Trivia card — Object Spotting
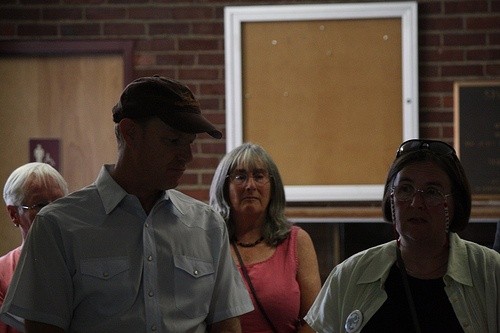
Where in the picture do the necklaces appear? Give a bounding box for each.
[233,232,266,248]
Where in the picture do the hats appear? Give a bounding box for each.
[112,74,223,139]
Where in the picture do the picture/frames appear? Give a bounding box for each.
[224,1,418,203]
[454,81,499,192]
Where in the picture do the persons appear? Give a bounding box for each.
[1,77,253,332]
[304,140,499,333]
[209,144,321,332]
[0,162,68,333]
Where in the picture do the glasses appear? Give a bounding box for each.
[394,139,464,184]
[226,172,272,185]
[16,202,49,213]
[391,185,456,207]
